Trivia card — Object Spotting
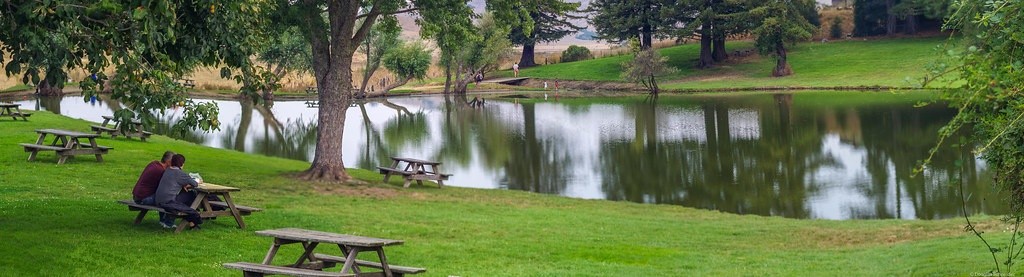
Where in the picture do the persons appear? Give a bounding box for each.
[545,81,547,89]
[133,151,202,229]
[555,81,558,90]
[513,62,519,78]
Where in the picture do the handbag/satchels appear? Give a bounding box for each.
[189,173,203,184]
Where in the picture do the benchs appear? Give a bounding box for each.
[89,125,118,131]
[20,143,71,151]
[313,253,426,273]
[174,82,196,86]
[118,201,189,215]
[14,112,31,115]
[306,89,318,92]
[419,171,454,177]
[223,262,358,277]
[208,201,263,212]
[74,143,114,150]
[129,128,154,134]
[377,167,411,175]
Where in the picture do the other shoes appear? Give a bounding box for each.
[159,222,177,229]
[190,224,201,230]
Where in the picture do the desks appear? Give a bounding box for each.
[244,228,406,277]
[172,183,246,234]
[0,103,27,121]
[96,116,146,142]
[172,76,194,90]
[28,129,103,164]
[383,157,443,188]
[307,87,318,93]
